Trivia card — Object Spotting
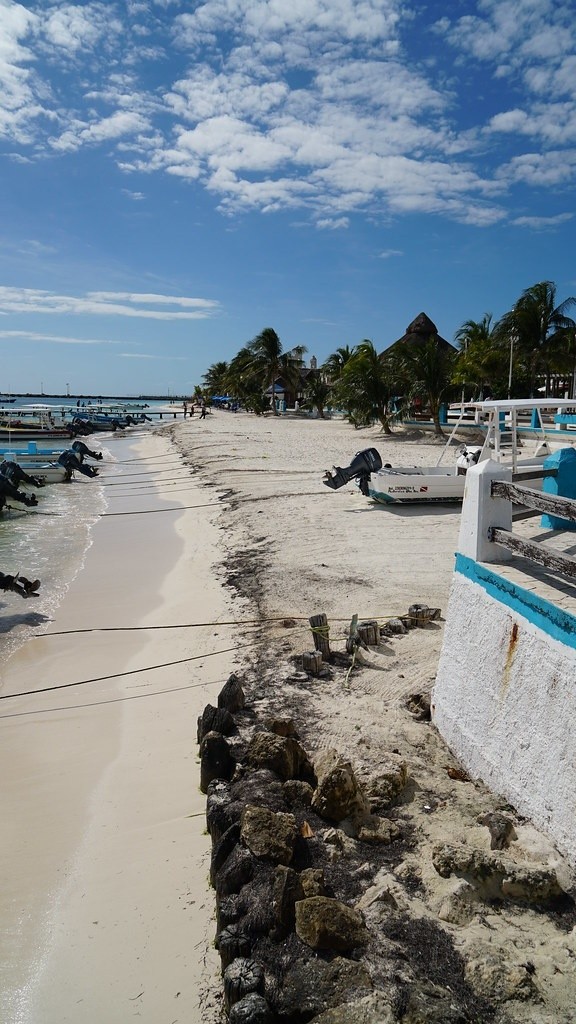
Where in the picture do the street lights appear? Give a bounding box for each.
[508,326,519,400]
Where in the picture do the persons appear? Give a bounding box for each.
[76,400,237,419]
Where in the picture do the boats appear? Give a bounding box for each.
[0,461,45,511]
[321,398,576,505]
[0,384,103,482]
[0,400,152,441]
[0,398,17,403]
